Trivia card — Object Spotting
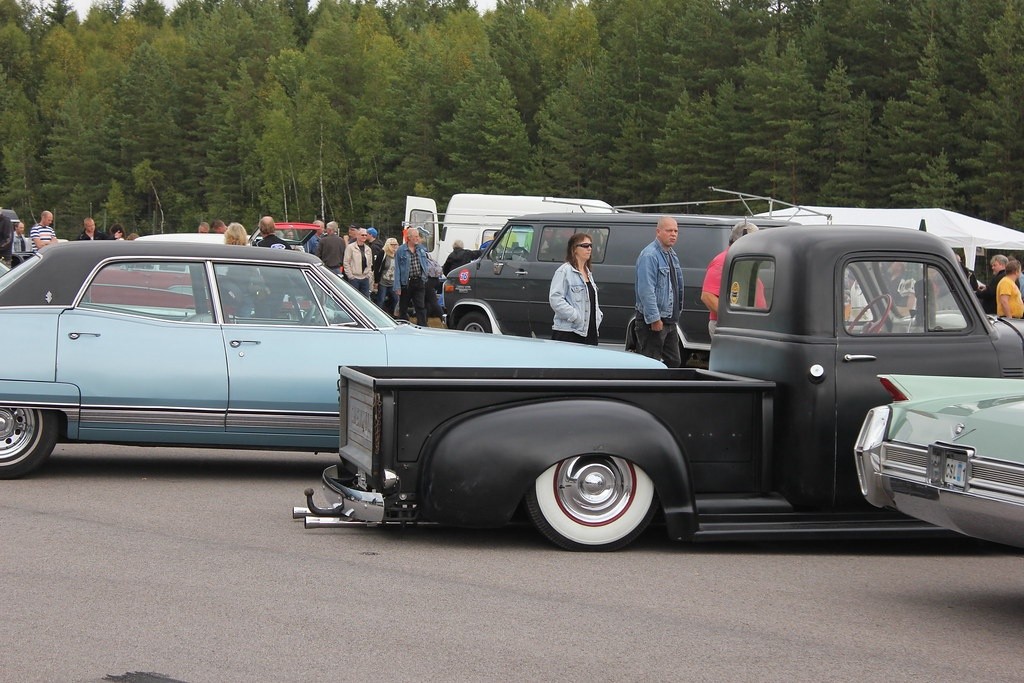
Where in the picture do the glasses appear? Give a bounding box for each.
[361,234,367,237]
[575,243,593,248]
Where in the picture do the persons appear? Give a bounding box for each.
[78,218,139,241]
[443,239,482,280]
[199,222,210,233]
[373,237,399,316]
[224,223,248,245]
[0,209,14,264]
[12,221,30,268]
[213,220,228,234]
[996,261,1024,319]
[254,216,292,250]
[303,220,384,300]
[635,217,684,368]
[30,210,58,255]
[394,228,428,327]
[1007,255,1024,300]
[701,222,767,338]
[845,255,978,321]
[549,233,603,346]
[978,254,1020,315]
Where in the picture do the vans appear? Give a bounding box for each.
[440,210,804,369]
[401,192,617,292]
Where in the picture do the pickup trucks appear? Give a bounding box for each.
[291,221,1024,552]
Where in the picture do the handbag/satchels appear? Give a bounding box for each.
[369,271,378,293]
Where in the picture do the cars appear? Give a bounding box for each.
[83,231,250,311]
[853,373,1024,555]
[248,221,345,313]
[0,239,672,481]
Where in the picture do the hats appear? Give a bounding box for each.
[366,228,378,238]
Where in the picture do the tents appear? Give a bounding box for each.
[755,206,1024,272]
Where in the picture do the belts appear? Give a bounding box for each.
[410,277,421,281]
